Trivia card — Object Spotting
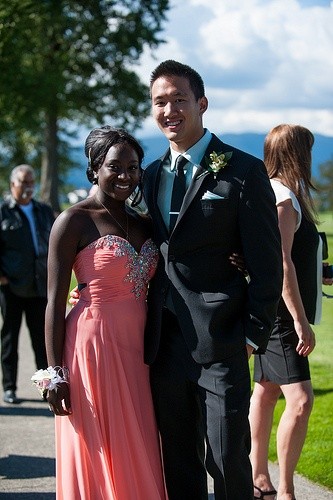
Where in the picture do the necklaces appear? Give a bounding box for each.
[94,192,131,245]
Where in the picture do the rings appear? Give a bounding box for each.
[308,344,312,347]
[299,340,303,344]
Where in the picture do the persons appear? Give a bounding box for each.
[246,124,333,500]
[69,59,283,500]
[0,161,62,405]
[32,125,250,500]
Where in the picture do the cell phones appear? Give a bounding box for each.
[323,265,333,278]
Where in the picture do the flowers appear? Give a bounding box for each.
[206,151,232,180]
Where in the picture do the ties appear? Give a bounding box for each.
[168,156,190,236]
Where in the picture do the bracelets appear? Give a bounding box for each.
[29,365,68,400]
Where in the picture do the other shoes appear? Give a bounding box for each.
[4,389,17,404]
[254,482,277,500]
